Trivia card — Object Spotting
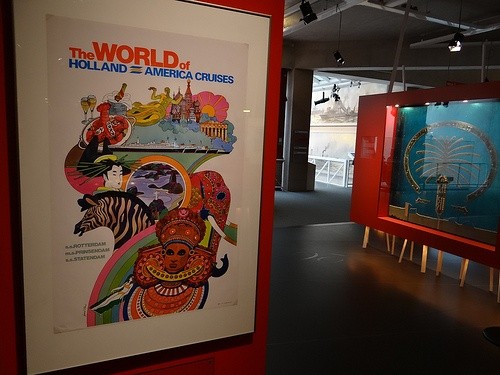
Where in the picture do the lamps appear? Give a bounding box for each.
[333,4,345,65]
[447,0,465,53]
[299,0,317,27]
